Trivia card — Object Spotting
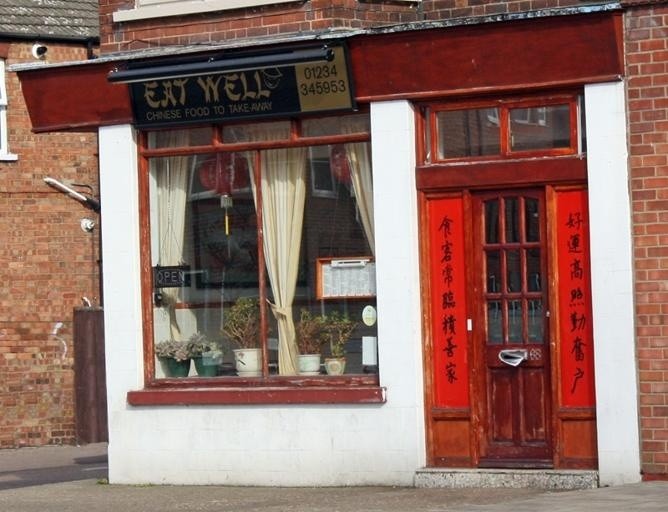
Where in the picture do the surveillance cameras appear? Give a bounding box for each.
[32,43,48,58]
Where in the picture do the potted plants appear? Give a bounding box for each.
[156,294,363,376]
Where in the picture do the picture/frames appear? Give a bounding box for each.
[314,255,376,303]
